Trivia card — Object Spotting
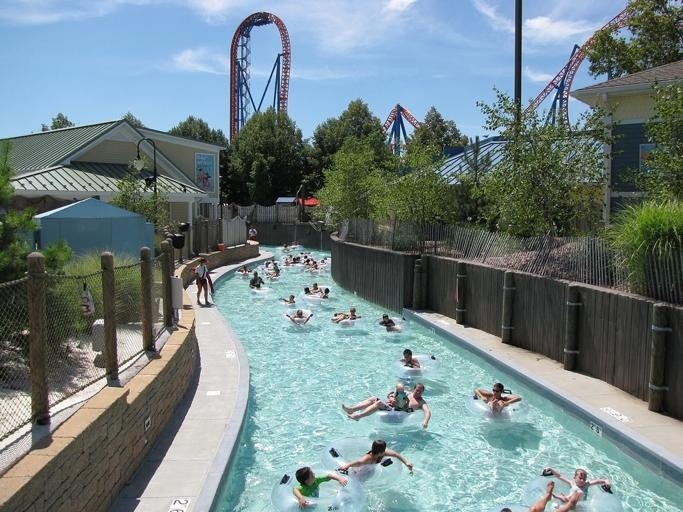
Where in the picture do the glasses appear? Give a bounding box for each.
[493,389,499,392]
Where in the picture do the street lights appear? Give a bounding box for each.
[133,136,158,235]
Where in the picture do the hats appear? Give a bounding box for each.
[199,259,207,263]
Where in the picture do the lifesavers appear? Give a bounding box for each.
[335,311,365,328]
[278,297,305,308]
[374,316,408,336]
[320,436,403,490]
[393,353,440,380]
[490,503,533,512]
[519,470,623,511]
[270,466,369,512]
[360,389,428,437]
[301,285,335,306]
[236,245,329,295]
[464,388,521,420]
[283,307,316,330]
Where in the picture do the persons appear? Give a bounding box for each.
[284,310,315,324]
[191,258,214,305]
[379,313,400,333]
[332,307,362,325]
[237,241,330,305]
[248,225,258,241]
